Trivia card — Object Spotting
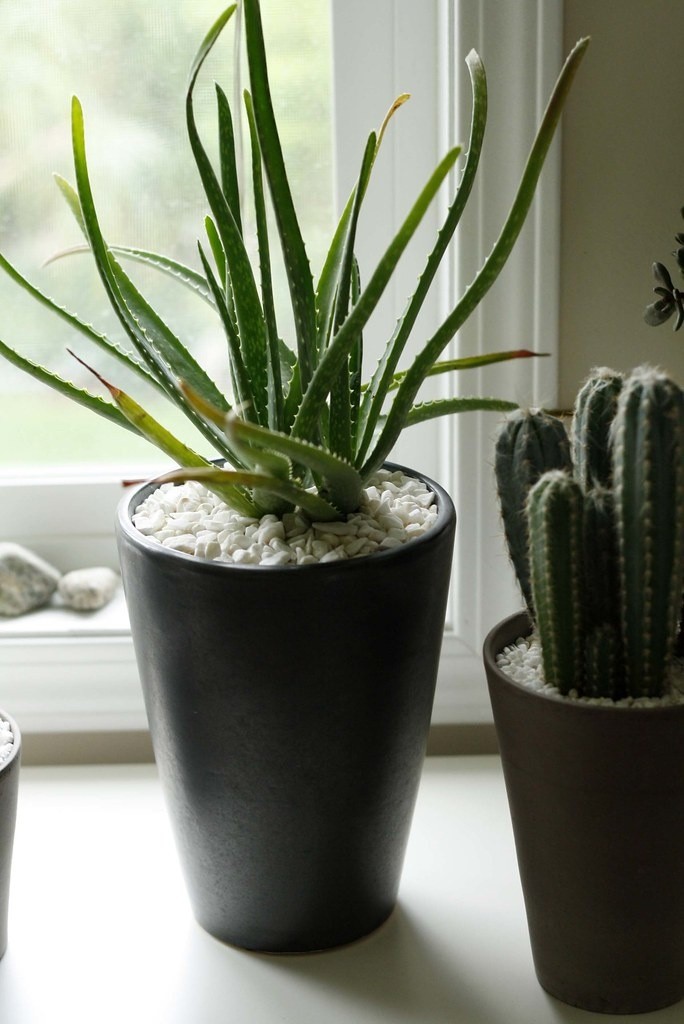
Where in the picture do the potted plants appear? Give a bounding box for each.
[476,351,684,1009]
[1,0,595,955]
